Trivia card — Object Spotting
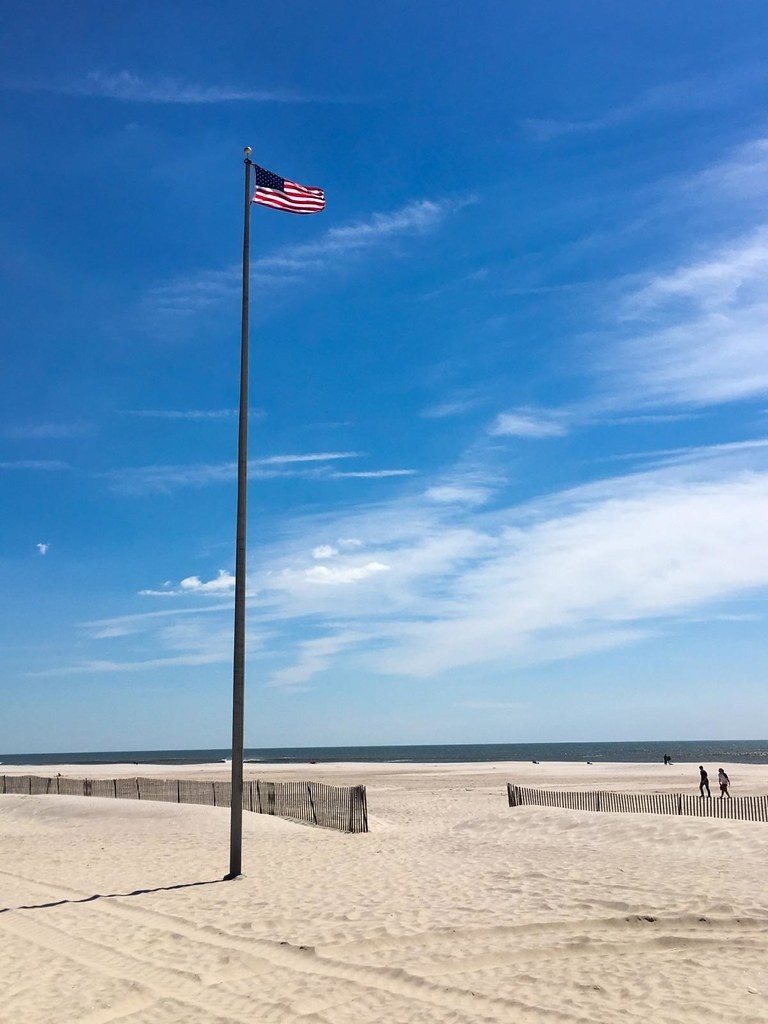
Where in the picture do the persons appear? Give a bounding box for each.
[699,765,711,798]
[663,753,672,765]
[718,768,732,800]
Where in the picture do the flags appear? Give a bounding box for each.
[250,162,326,215]
